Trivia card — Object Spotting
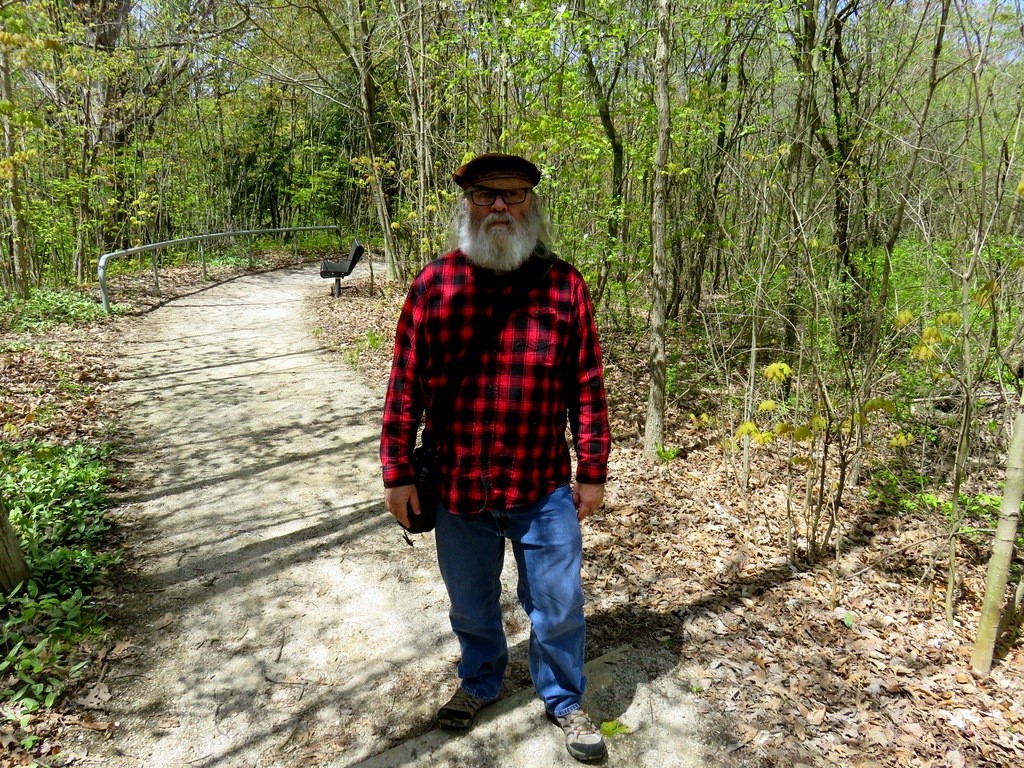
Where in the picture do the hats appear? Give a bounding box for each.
[452,154,541,195]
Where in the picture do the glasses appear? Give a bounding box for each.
[466,188,534,206]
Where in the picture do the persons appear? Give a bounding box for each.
[380,151,612,763]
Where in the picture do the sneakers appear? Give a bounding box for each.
[437,686,504,728]
[546,708,604,760]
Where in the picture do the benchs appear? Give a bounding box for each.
[319,238,365,298]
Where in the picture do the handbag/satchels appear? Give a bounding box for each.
[396,447,437,534]
